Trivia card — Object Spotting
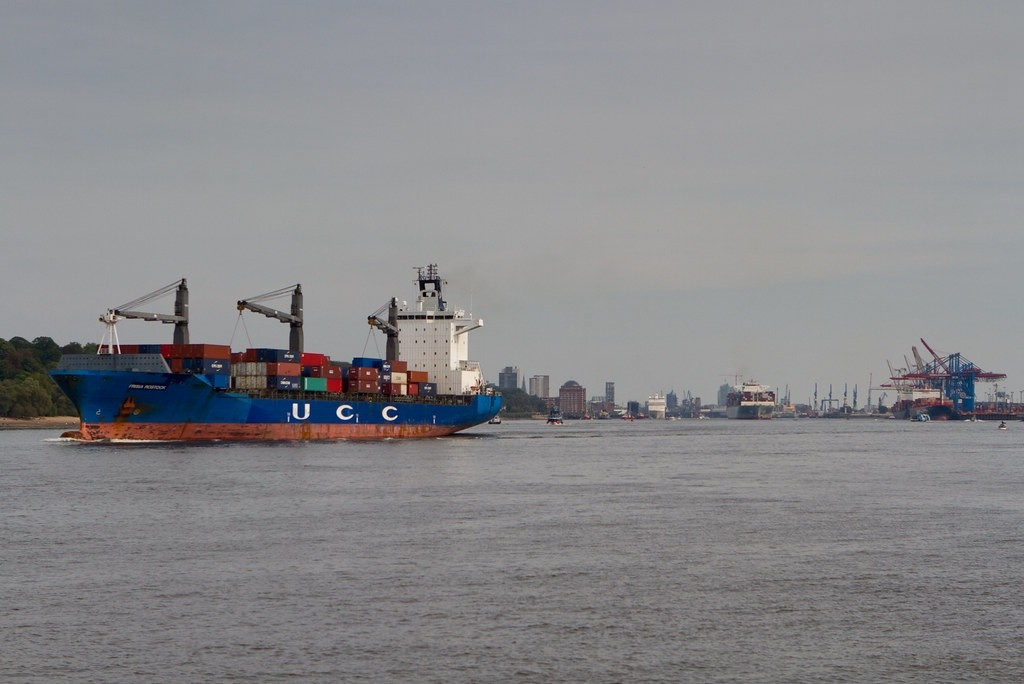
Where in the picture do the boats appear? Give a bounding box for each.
[726,378,775,420]
[891,399,916,420]
[597,410,610,419]
[912,411,930,422]
[488,416,502,424]
[799,414,808,417]
[909,397,954,420]
[696,413,710,420]
[964,414,984,423]
[547,404,564,424]
[772,403,796,418]
[583,414,590,419]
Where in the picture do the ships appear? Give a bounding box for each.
[49,263,506,439]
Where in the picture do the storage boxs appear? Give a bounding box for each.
[97,343,438,397]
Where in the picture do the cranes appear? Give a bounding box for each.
[722,373,744,386]
